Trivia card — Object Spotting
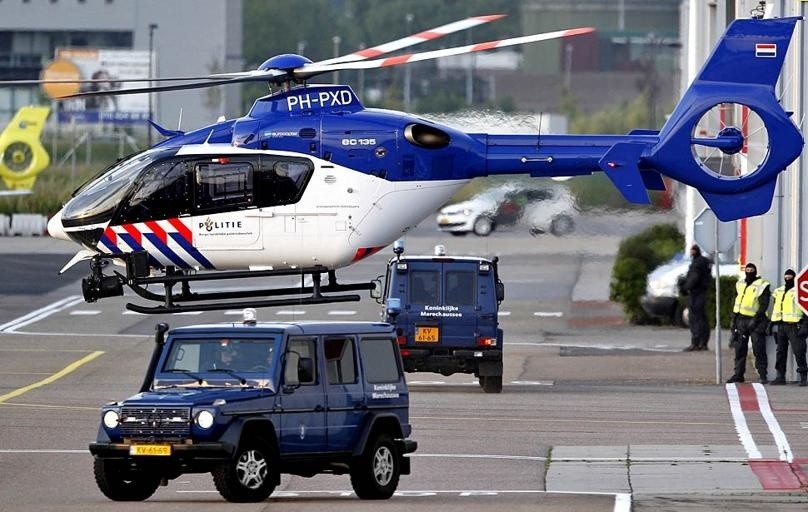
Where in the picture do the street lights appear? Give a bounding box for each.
[148,22,159,147]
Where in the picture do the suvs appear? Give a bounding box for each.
[370,240,504,393]
[84,320,417,503]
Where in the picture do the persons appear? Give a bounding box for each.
[726,263,772,384]
[676,244,717,352]
[83,69,119,111]
[197,340,242,373]
[768,267,808,387]
[261,345,312,385]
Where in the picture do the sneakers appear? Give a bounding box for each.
[797,379,807,387]
[755,375,768,384]
[725,373,744,383]
[769,376,787,387]
[682,344,709,352]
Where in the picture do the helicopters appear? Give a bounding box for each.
[1,15,803,315]
[0,105,51,191]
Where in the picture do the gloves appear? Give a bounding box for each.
[765,321,773,336]
[729,316,737,331]
[796,319,808,339]
[746,319,757,331]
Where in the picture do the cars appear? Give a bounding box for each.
[638,232,741,329]
[436,183,578,236]
